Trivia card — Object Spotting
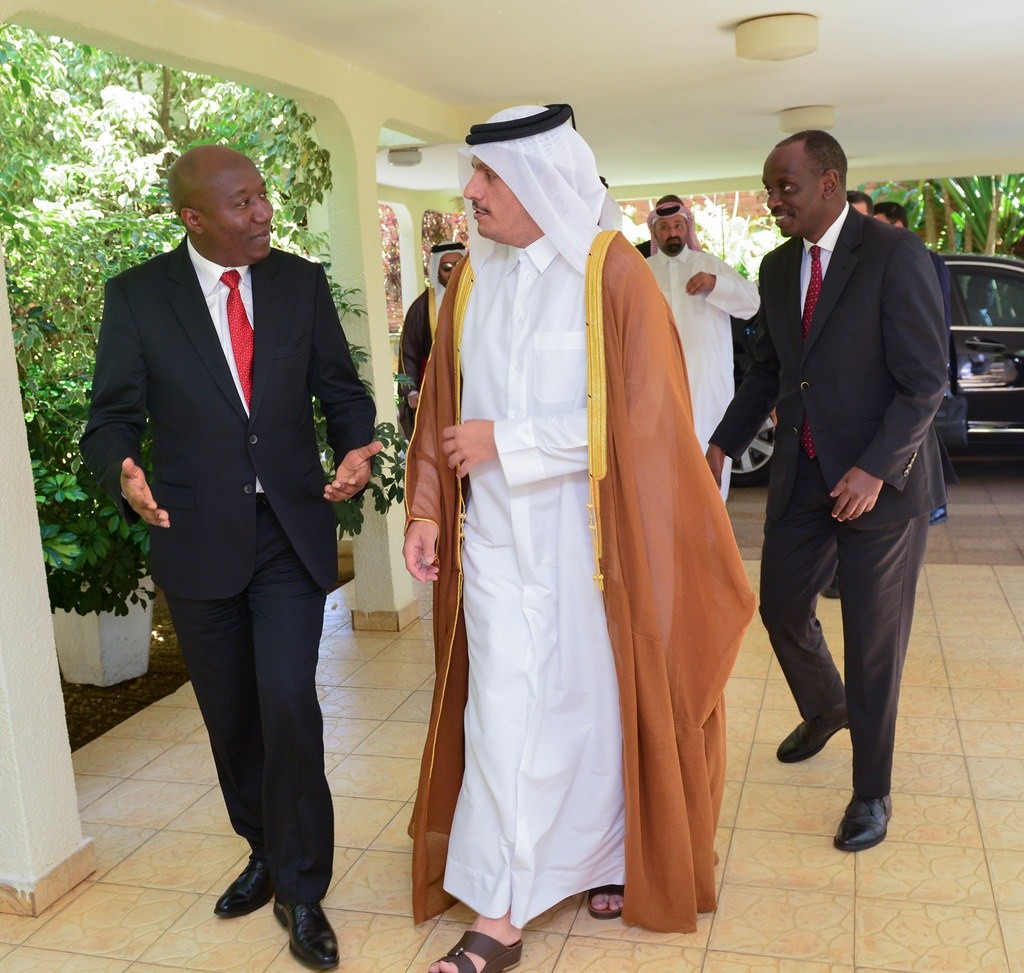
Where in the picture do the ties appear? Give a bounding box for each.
[220,270,255,410]
[801,243,824,462]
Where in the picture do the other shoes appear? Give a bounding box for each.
[928,503,947,525]
[820,570,840,598]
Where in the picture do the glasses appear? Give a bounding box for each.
[439,260,457,272]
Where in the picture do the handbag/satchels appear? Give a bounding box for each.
[932,387,971,447]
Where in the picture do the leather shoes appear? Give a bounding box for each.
[214,858,274,919]
[776,715,850,763]
[272,900,339,969]
[833,793,893,851]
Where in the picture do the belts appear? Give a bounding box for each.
[257,494,268,505]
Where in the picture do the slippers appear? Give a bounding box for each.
[425,931,523,973]
[588,883,626,920]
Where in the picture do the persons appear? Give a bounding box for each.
[400,106,754,973]
[819,191,953,599]
[641,193,762,508]
[967,275,998,326]
[78,145,384,971]
[707,128,950,853]
[398,241,468,440]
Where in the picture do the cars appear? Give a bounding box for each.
[728,253,1024,485]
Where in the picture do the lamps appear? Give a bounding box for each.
[779,104,839,134]
[736,14,818,60]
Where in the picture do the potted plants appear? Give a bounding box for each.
[9,306,154,687]
